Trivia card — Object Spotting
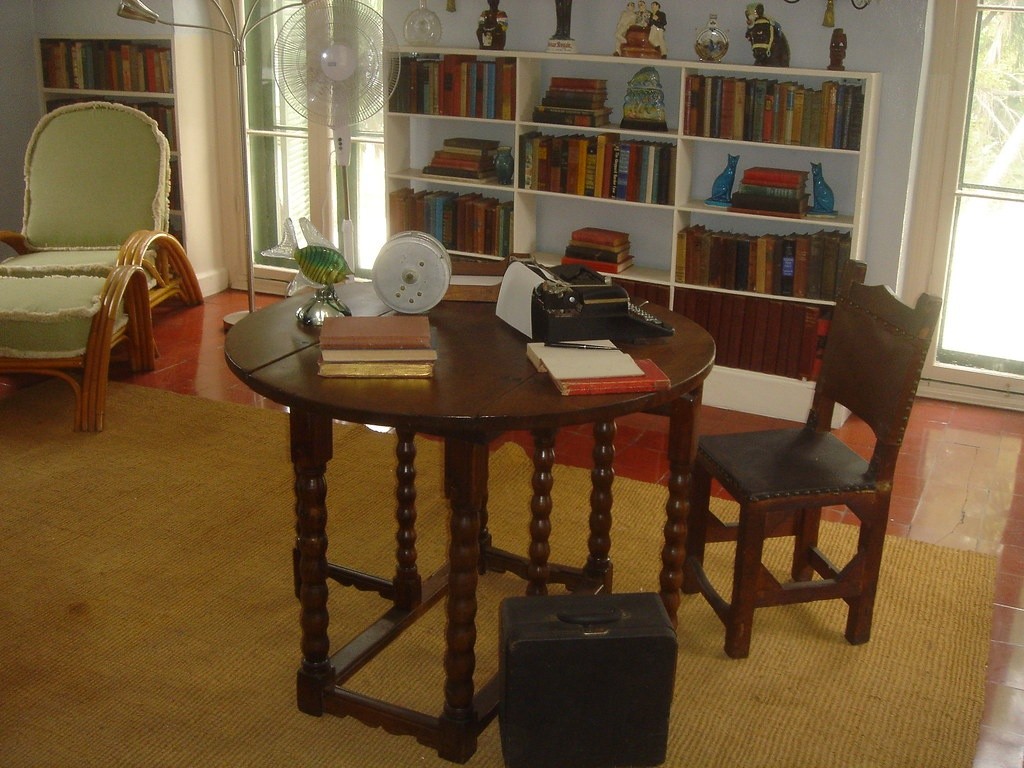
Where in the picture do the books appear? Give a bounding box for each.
[674,289,834,382]
[422,138,500,184]
[526,339,671,396]
[318,317,436,377]
[41,39,173,93]
[390,188,514,263]
[728,167,811,218]
[561,227,634,275]
[532,77,613,127]
[47,96,183,246]
[613,278,670,309]
[519,131,677,206]
[388,55,516,121]
[675,225,851,302]
[684,75,865,151]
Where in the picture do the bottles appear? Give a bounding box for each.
[404,1,441,48]
[493,146,513,185]
[695,14,728,63]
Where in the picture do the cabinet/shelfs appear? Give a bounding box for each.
[381,47,882,431]
[34,35,229,298]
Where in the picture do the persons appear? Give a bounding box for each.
[613,1,667,58]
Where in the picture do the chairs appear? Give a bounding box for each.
[683,258,943,661]
[0,101,205,433]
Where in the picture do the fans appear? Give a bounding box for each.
[273,0,403,317]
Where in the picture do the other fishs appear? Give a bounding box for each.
[261,217,354,296]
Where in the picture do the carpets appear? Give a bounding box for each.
[0,378,1007,768]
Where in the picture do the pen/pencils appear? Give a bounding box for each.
[544,342,619,351]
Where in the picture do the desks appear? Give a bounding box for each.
[228,282,717,765]
